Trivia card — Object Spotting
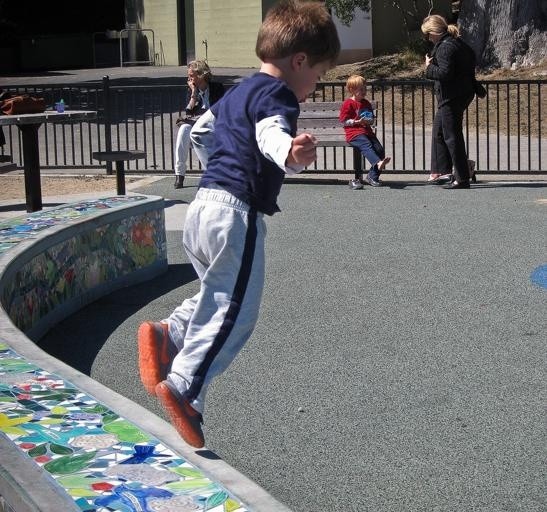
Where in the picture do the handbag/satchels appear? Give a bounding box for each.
[0,91,45,115]
[176,109,207,127]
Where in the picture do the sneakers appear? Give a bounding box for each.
[138,322,178,397]
[155,378,205,448]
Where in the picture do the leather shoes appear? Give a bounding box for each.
[349,179,363,189]
[174,175,184,188]
[430,175,454,185]
[367,174,383,186]
[443,180,469,189]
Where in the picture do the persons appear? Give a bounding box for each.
[421,15,475,189]
[139,0,341,448]
[339,74,390,187]
[173,61,225,188]
